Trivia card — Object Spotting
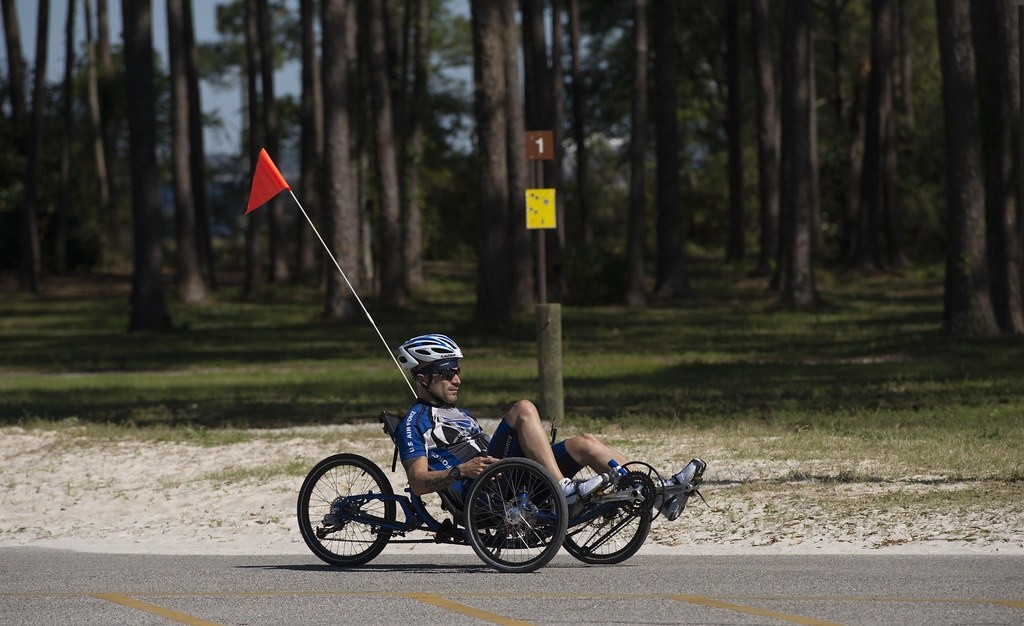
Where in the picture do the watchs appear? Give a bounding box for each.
[450,465,462,482]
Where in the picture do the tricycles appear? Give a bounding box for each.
[294,412,705,573]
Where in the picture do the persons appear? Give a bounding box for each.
[398,333,707,521]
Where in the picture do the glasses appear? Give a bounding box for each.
[431,367,460,380]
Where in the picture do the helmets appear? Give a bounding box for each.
[397,334,463,369]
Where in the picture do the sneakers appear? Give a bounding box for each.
[655,458,704,522]
[553,473,609,520]
